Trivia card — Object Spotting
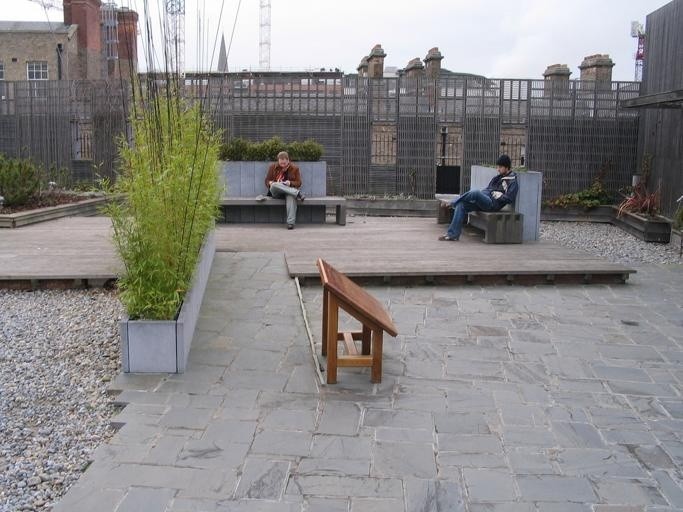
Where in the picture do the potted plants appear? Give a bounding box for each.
[467,162,672,242]
[93,1,242,375]
[215,135,325,223]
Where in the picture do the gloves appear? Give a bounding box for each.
[491,192,503,200]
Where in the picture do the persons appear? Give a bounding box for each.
[265,152,305,229]
[439,155,518,240]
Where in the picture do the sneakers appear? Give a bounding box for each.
[299,191,305,201]
[438,234,457,241]
[288,225,294,229]
[441,202,453,209]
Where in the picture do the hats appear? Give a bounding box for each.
[496,155,511,168]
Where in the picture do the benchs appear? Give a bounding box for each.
[216,195,346,226]
[435,193,524,244]
